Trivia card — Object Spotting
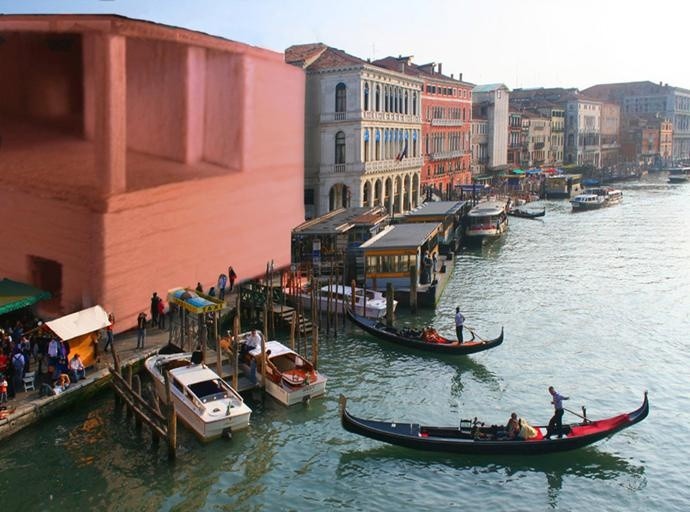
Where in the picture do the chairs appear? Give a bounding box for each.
[459,419,472,435]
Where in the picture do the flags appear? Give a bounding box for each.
[396,138,403,161]
[399,138,408,161]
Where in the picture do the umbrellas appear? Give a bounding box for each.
[512,162,564,175]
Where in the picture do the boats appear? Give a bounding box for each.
[465,192,547,239]
[282,282,398,320]
[668,174,688,182]
[569,186,623,211]
[343,391,649,457]
[346,307,505,356]
[144,328,328,444]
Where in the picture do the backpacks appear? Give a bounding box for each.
[13,354,23,368]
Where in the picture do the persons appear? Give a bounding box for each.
[421,249,439,283]
[497,412,521,441]
[239,328,259,365]
[453,306,465,344]
[250,349,277,385]
[543,386,569,439]
[0,263,237,404]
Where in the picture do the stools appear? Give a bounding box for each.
[22,376,36,393]
[25,370,35,378]
